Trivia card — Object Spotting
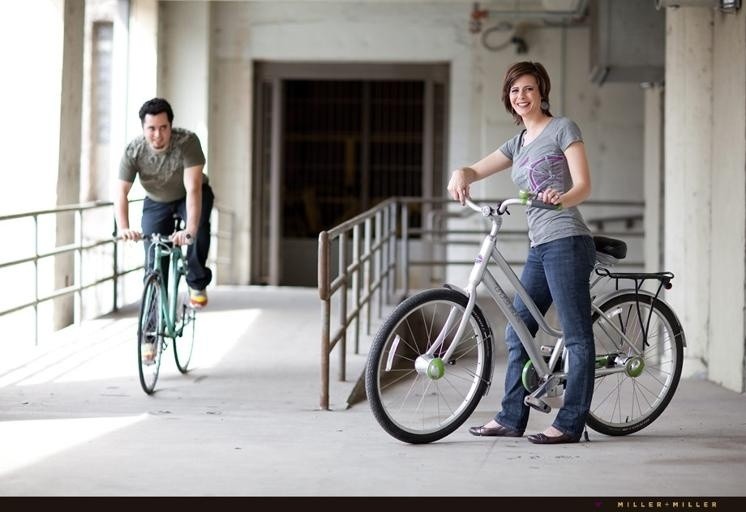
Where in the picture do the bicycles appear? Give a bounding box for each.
[112,234,198,396]
[364,196,687,446]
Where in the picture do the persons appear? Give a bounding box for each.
[113,98,215,366]
[446,60,603,447]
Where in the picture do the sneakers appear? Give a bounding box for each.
[189,287,208,308]
[142,343,157,362]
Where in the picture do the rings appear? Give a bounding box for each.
[555,194,560,199]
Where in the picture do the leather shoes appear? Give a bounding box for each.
[468,424,523,438]
[527,433,580,445]
